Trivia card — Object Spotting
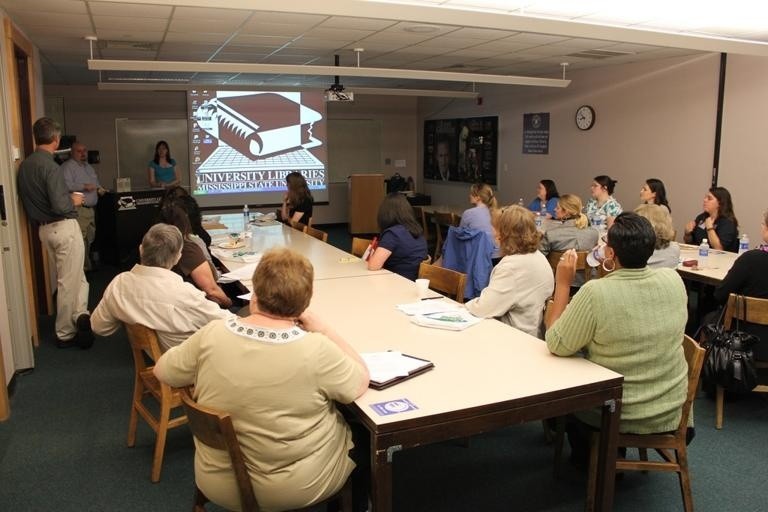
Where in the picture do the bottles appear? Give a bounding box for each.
[539,204,547,217]
[697,238,710,269]
[384,180,388,196]
[534,212,543,228]
[585,246,611,267]
[519,198,525,207]
[243,204,249,223]
[591,212,601,233]
[738,232,751,257]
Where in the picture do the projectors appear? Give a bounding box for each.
[323,85,354,103]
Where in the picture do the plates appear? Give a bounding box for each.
[219,240,247,249]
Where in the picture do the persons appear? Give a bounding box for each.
[364,191,694,475]
[17,115,94,351]
[89,190,374,511]
[454,126,469,175]
[60,142,106,276]
[147,141,181,188]
[526,174,768,372]
[280,172,313,227]
[428,134,460,181]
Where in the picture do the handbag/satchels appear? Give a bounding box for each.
[699,294,759,392]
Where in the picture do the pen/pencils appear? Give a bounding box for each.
[421,296,444,300]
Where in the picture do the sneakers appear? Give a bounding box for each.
[78,314,93,350]
[57,340,76,349]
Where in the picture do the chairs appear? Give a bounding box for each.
[177,389,258,512]
[128,325,194,482]
[128,208,767,344]
[617,333,707,512]
[714,292,767,430]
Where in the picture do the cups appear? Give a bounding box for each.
[414,278,430,296]
[73,192,83,195]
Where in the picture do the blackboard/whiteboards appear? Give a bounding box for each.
[115,118,384,191]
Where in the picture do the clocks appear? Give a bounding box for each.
[576,106,595,131]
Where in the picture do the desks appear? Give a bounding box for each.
[200,209,625,512]
